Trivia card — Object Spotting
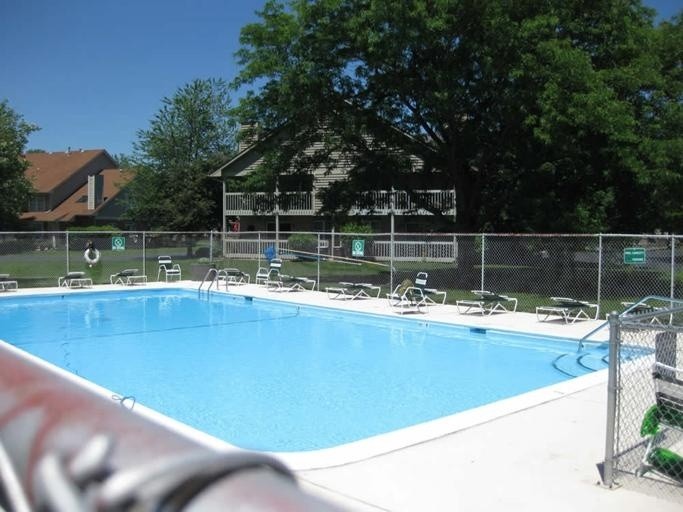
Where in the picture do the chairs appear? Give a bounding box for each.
[157,256,181,284]
[110,268,148,287]
[58,271,93,290]
[636,361,683,488]
[0,274,18,293]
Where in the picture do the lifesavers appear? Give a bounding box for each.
[85,247,101,264]
